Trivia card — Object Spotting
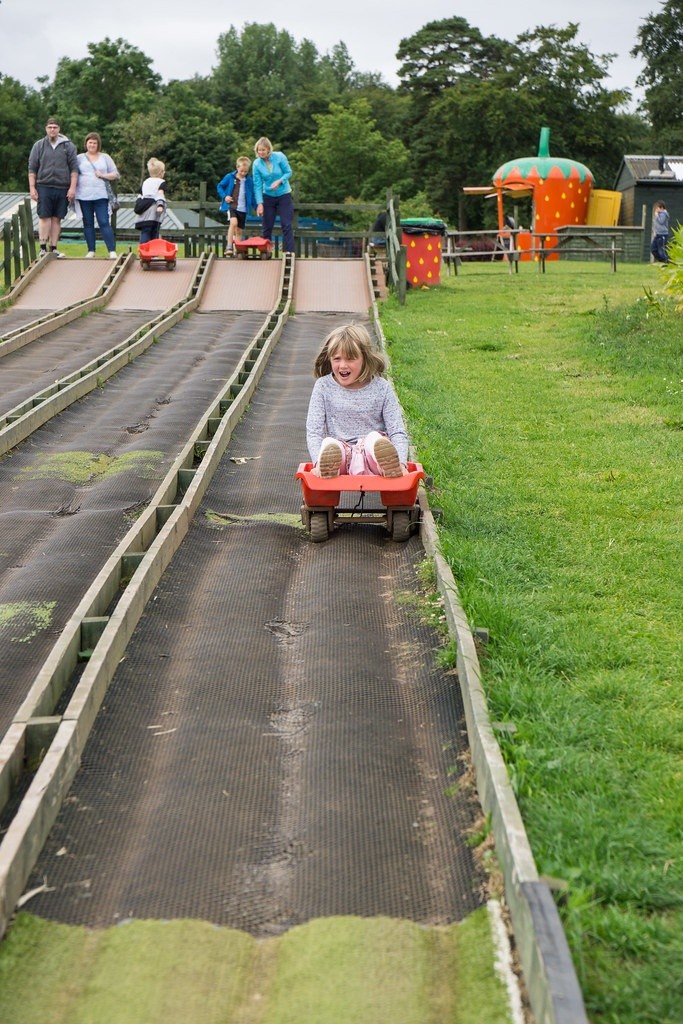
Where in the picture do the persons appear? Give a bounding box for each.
[135,157,168,245]
[252,136,295,252]
[650,199,669,264]
[217,156,258,255]
[28,118,78,258]
[75,132,121,258]
[306,325,409,478]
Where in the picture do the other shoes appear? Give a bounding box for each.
[374,438,401,477]
[224,247,233,255]
[268,252,272,258]
[319,443,342,478]
[53,250,65,257]
[86,252,95,258]
[39,250,45,257]
[110,251,117,259]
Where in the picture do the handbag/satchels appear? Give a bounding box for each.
[106,183,120,212]
[134,198,155,214]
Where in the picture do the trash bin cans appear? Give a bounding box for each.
[401,217,448,287]
